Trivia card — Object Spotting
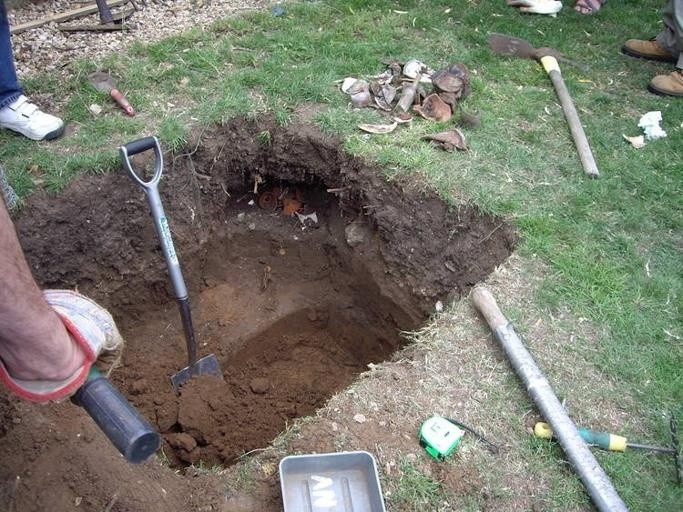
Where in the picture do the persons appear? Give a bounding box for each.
[0,170,125,404]
[623,1,683,99]
[1,0,65,141]
[573,0,606,15]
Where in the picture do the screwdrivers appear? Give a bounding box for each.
[534,421,676,453]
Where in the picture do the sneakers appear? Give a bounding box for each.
[648,69,683,96]
[0,94,65,141]
[622,36,678,62]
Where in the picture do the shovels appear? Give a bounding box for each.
[119,136,225,397]
[88,72,135,118]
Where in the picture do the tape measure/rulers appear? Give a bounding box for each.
[418,414,465,463]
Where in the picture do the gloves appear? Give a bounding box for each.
[0,289,124,402]
[507,0,563,14]
